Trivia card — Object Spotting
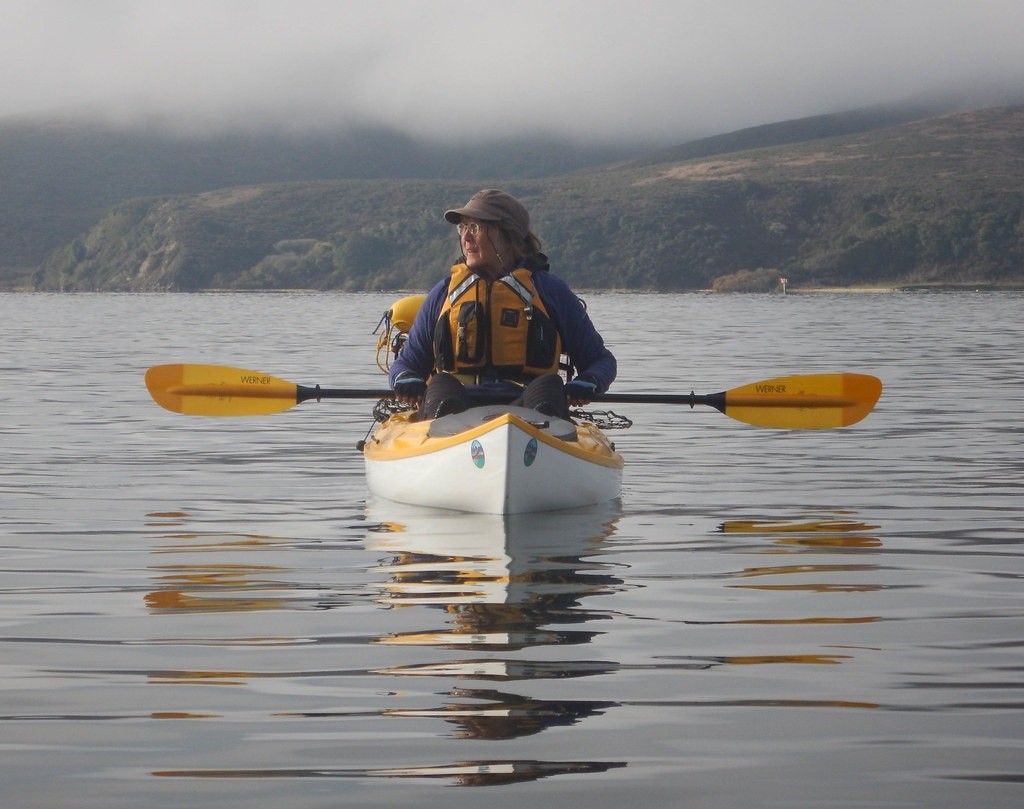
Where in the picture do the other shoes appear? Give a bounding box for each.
[434,396,467,420]
[534,402,556,416]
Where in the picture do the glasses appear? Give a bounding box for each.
[456,222,502,238]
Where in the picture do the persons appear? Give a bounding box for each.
[389,188,618,418]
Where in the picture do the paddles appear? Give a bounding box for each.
[143,363,885,434]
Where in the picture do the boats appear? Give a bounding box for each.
[361,405,628,518]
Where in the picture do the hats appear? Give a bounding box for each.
[444,188,529,239]
